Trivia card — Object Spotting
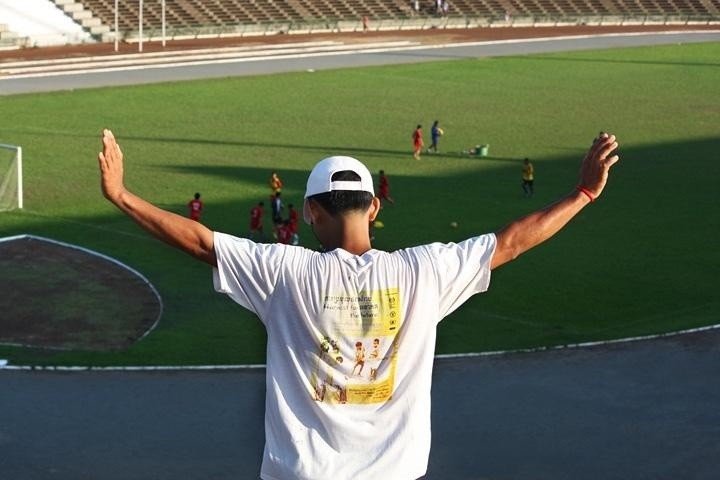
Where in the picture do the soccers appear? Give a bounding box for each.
[450,221,458,228]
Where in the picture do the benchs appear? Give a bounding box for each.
[76,0,720,30]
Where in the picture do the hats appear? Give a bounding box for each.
[302,156,376,226]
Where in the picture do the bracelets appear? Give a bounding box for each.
[574,182,595,205]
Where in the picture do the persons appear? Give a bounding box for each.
[246,164,393,254]
[426,120,440,156]
[185,192,203,221]
[520,158,535,198]
[412,125,424,161]
[95,124,620,480]
